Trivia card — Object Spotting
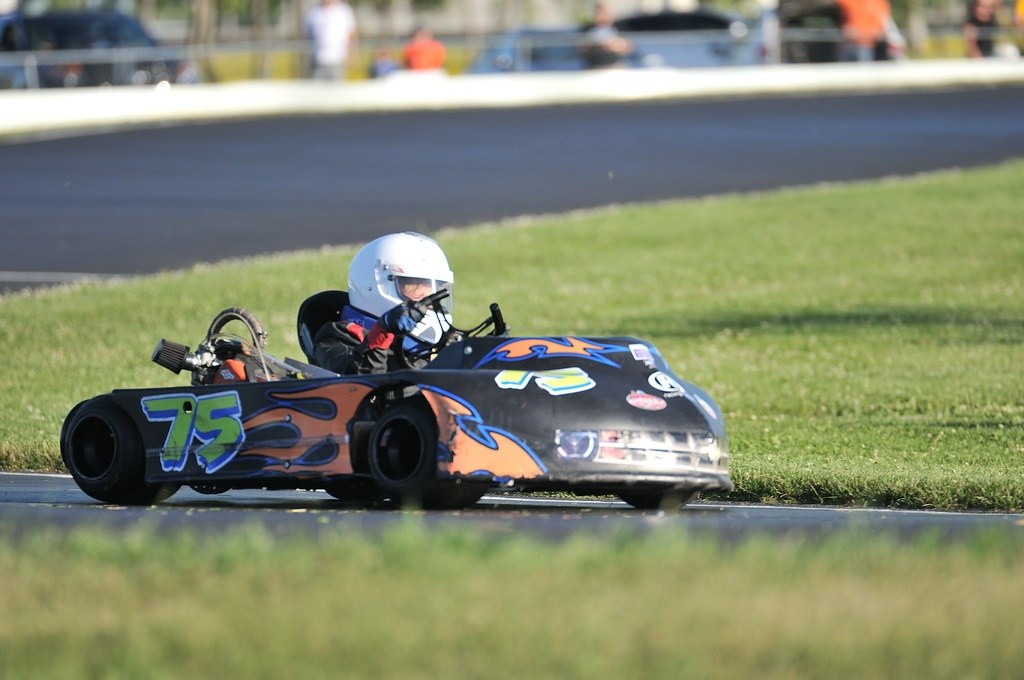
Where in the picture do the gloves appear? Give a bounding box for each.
[379,299,427,333]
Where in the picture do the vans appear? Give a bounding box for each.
[0,8,205,93]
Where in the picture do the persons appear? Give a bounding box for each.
[575,5,630,67]
[962,0,1005,57]
[403,27,445,67]
[302,0,358,80]
[311,231,456,372]
[837,0,903,63]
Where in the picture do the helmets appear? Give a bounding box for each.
[346,230,455,346]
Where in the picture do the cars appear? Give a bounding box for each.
[475,9,907,72]
